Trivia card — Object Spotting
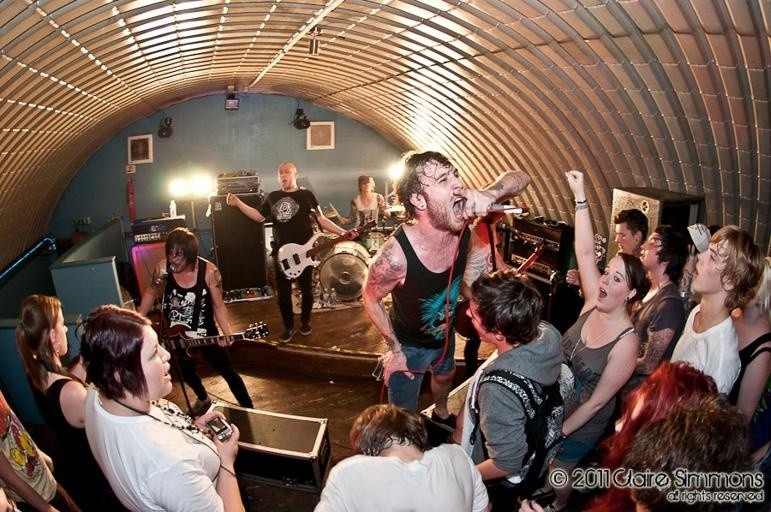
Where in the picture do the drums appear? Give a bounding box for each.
[318,240,373,301]
[366,226,395,253]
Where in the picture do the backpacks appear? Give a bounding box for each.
[470,371,565,493]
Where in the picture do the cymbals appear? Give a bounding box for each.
[385,205,406,212]
[309,209,337,219]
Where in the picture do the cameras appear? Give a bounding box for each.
[205,416,233,442]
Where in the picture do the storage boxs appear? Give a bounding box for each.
[203,400,332,494]
[419,375,472,448]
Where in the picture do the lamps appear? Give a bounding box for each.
[222,87,240,112]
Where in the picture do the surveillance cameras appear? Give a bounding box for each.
[293,108,310,130]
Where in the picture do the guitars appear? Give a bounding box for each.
[277,220,377,279]
[152,320,269,362]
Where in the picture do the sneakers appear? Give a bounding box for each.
[430,407,457,430]
[298,315,312,336]
[185,391,210,415]
[277,324,297,343]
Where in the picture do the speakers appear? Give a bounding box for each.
[607,186,706,269]
[210,192,265,291]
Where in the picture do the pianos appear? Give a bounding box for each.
[508,215,575,323]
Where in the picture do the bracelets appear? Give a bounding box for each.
[220,463,238,477]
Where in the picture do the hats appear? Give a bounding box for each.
[687,223,712,253]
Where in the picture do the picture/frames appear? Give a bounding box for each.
[126,132,153,165]
[304,119,336,151]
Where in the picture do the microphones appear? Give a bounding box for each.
[457,199,528,214]
[154,264,175,285]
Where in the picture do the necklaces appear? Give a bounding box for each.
[113,391,201,431]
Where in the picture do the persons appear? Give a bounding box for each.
[14,290,129,511]
[76,302,249,511]
[0,478,23,512]
[225,161,362,344]
[314,149,770,511]
[0,390,82,511]
[135,225,253,418]
